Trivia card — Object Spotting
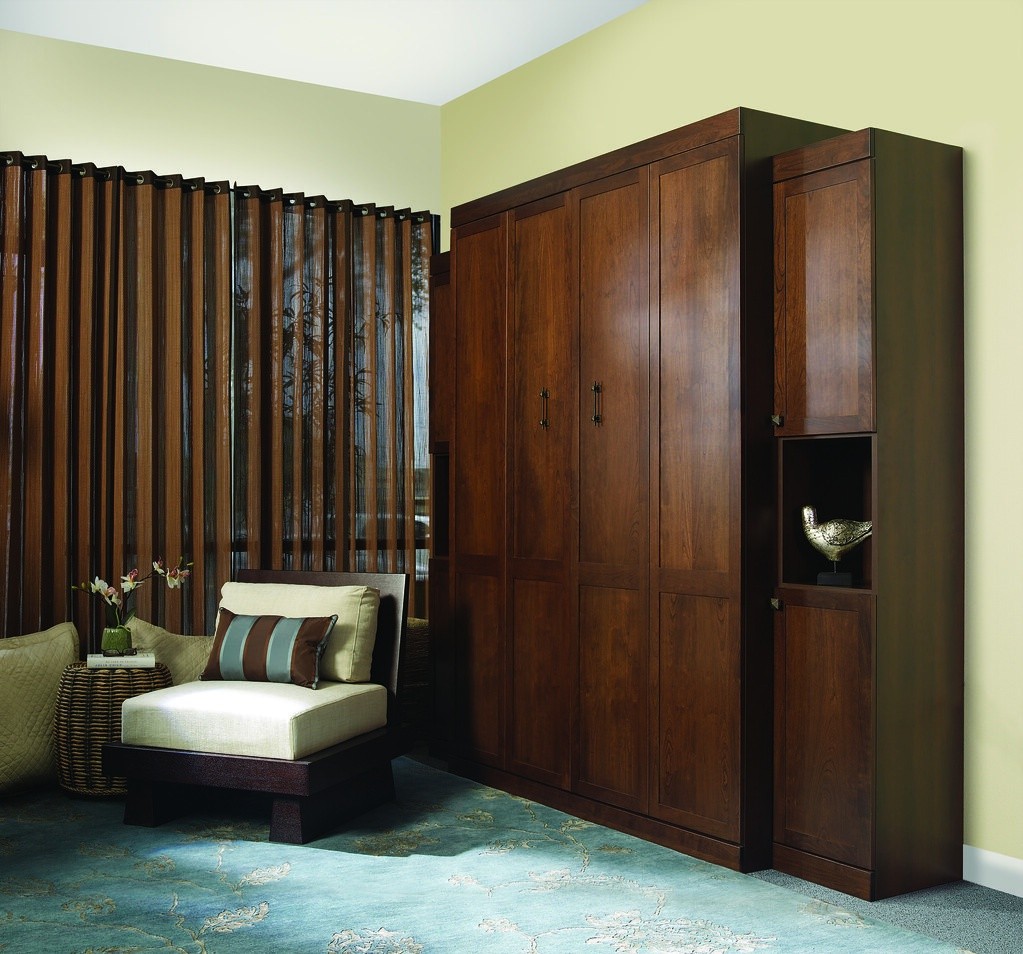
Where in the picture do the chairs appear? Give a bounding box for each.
[102,568,411,847]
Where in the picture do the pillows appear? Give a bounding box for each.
[200,608,339,692]
[0,621,80,796]
[218,580,380,684]
[124,616,216,687]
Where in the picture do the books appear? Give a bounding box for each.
[86,652,155,668]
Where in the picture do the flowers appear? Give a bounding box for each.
[70,556,194,633]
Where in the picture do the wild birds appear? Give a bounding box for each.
[801,505,872,561]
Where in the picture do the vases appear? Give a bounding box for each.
[101,627,137,657]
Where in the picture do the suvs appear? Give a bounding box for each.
[231,514,430,581]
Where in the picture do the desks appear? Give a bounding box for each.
[52,662,173,800]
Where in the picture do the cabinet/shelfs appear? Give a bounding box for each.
[427,106,968,909]
